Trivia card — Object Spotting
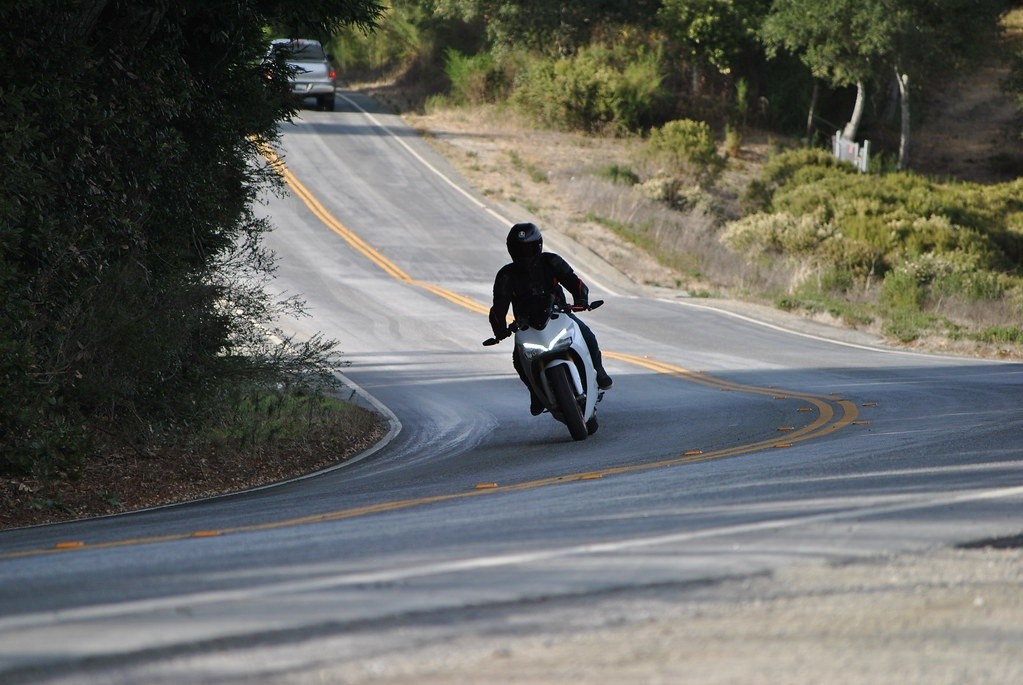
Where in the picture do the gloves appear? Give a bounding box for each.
[495,328,511,340]
[573,299,587,312]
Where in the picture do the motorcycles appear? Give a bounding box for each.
[482,300,606,441]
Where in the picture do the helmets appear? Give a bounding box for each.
[507,223,543,272]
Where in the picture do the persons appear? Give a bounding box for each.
[489,223,614,416]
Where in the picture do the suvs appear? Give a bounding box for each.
[256,38,337,111]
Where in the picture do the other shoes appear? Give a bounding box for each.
[530,390,545,416]
[595,367,613,390]
